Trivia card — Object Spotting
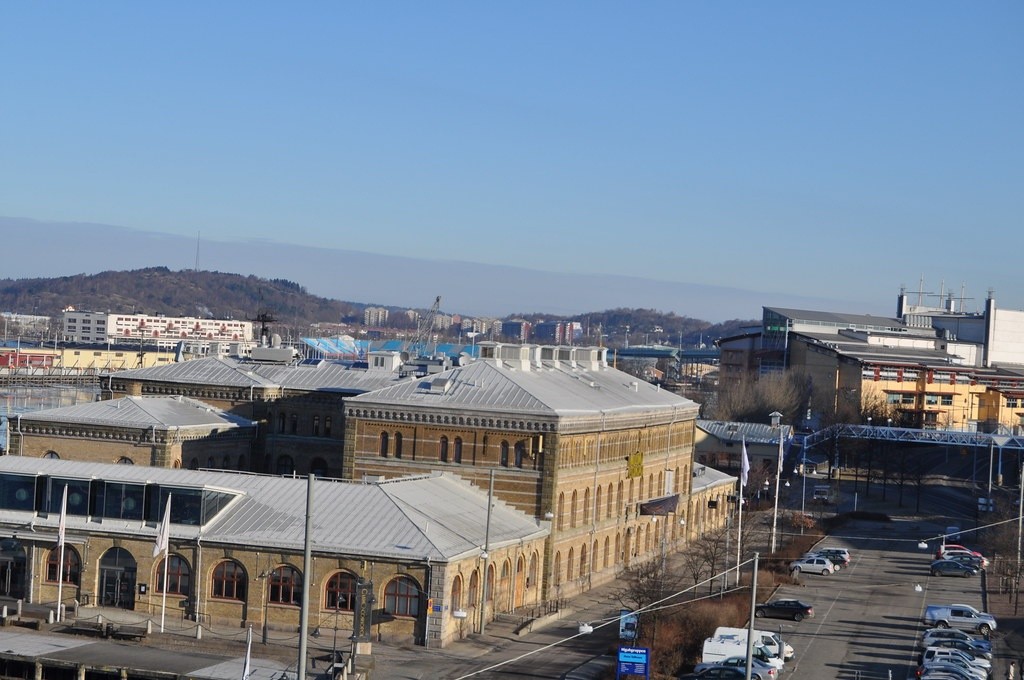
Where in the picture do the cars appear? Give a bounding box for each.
[680,544,997,680]
[944,526,961,545]
[755,598,816,622]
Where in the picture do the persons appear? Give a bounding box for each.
[1007,661,1016,680]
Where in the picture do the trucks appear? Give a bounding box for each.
[813,485,834,506]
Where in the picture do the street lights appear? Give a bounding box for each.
[713,490,747,590]
[307,609,360,680]
[335,576,378,655]
[794,465,817,534]
[652,511,685,599]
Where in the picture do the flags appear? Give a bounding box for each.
[152,494,171,556]
[779,431,785,474]
[57,485,68,547]
[742,438,750,488]
